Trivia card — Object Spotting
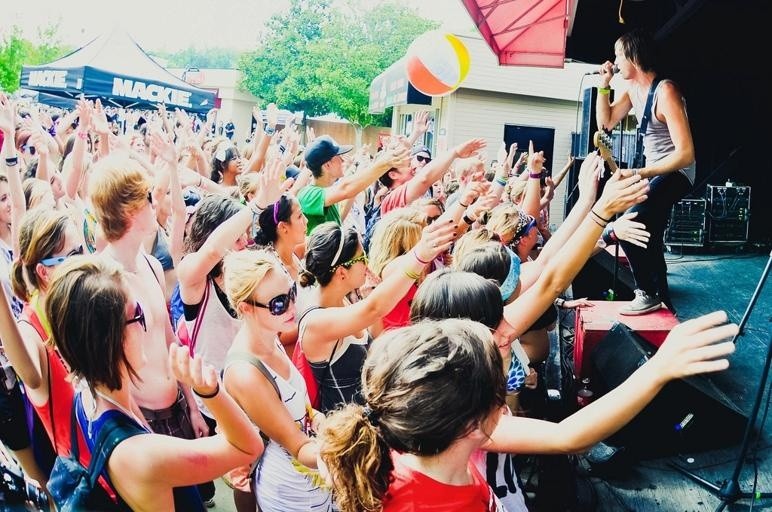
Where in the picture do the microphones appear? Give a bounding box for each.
[584,66,619,76]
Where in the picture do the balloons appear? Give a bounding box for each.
[406,29,471,97]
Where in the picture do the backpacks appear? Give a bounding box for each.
[33,393,144,512]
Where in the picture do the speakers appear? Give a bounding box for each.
[580,319,749,481]
[563,156,626,221]
[578,88,612,155]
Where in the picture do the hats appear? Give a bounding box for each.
[413,146,431,155]
[304,134,353,170]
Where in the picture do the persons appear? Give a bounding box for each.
[0,85,738,512]
[595,32,697,317]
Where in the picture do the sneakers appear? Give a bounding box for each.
[619,289,662,317]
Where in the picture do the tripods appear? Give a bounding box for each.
[665,328,771,511]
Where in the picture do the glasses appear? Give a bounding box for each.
[417,155,431,163]
[244,281,297,315]
[525,214,537,234]
[39,245,86,268]
[329,251,369,272]
[123,302,147,332]
